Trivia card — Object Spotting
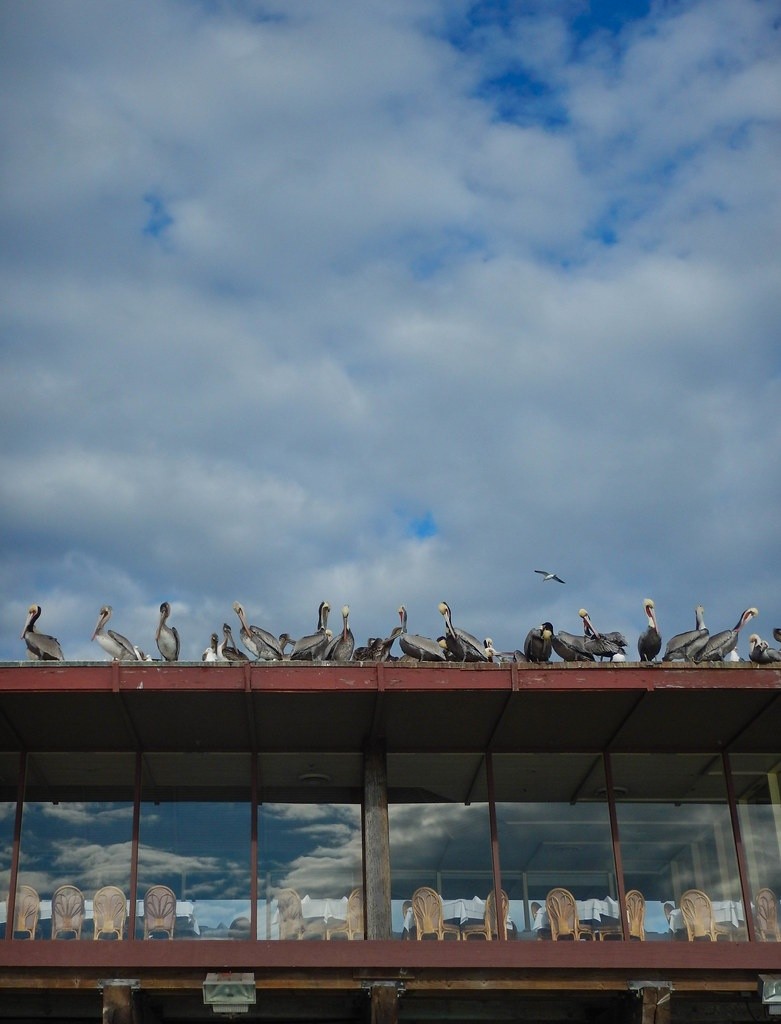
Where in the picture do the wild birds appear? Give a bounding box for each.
[398,605,447,662]
[202,633,220,662]
[534,570,565,584]
[20,605,64,662]
[323,605,355,661]
[216,623,249,660]
[278,601,333,661]
[434,602,502,663]
[512,597,781,664]
[153,602,180,663]
[233,600,284,662]
[90,605,146,662]
[353,627,402,662]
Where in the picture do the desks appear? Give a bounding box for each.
[404,896,513,940]
[531,896,629,942]
[271,895,348,940]
[0,900,200,939]
[668,900,755,941]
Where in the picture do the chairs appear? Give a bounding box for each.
[461,889,509,940]
[531,902,551,941]
[92,886,127,940]
[326,888,363,940]
[664,903,685,941]
[4,886,39,940]
[680,889,735,942]
[52,885,85,940]
[597,890,645,941]
[546,887,596,942]
[274,889,325,941]
[736,888,781,941]
[411,887,460,940]
[143,885,176,940]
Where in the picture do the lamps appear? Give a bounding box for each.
[203,979,257,1005]
